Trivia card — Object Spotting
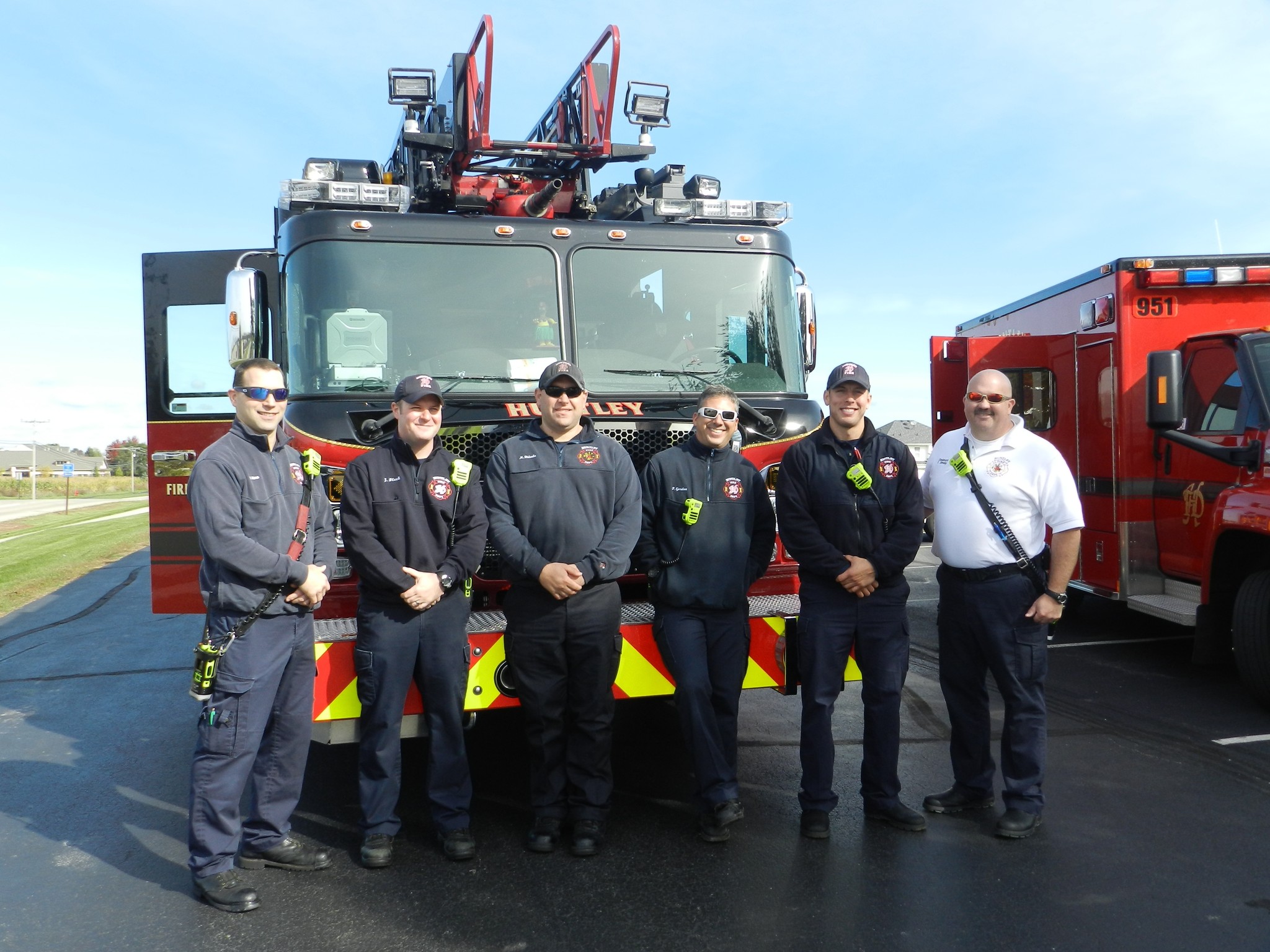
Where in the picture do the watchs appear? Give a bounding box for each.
[1044,588,1068,604]
[436,572,452,590]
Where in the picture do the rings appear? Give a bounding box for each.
[415,601,420,605]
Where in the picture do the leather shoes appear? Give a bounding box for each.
[361,832,393,867]
[238,830,332,871]
[444,828,476,858]
[192,867,261,913]
[922,785,997,814]
[995,808,1042,837]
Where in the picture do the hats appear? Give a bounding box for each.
[826,360,871,393]
[395,374,446,406]
[539,360,586,391]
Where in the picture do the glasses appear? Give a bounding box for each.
[543,386,582,398]
[233,385,290,401]
[698,407,738,421]
[967,393,1011,404]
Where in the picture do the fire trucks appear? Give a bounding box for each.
[140,16,863,792]
[929,252,1270,721]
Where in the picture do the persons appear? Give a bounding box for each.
[486,362,642,855]
[637,384,776,843]
[341,375,489,867]
[920,369,1086,838]
[185,357,337,912]
[777,362,928,838]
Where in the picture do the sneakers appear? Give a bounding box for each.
[529,818,562,853]
[716,797,743,824]
[570,817,602,854]
[865,798,926,831]
[800,807,829,838]
[697,804,728,840]
